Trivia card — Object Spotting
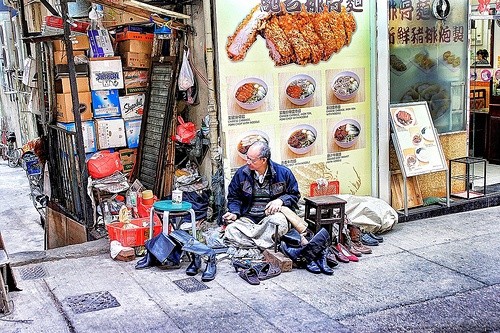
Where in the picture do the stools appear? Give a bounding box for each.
[304,195,347,245]
[149,200,197,262]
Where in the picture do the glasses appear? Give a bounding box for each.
[243,155,262,164]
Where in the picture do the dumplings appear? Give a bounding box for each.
[401,83,450,119]
[443,51,461,67]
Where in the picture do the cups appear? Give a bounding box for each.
[142,189,154,205]
[171,190,182,204]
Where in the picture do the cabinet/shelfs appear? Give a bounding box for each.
[449,157,486,200]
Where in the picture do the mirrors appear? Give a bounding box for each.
[470,8,495,68]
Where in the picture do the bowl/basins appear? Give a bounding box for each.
[286,125,319,154]
[285,74,316,105]
[332,70,361,100]
[232,78,268,110]
[331,119,362,148]
[236,129,269,161]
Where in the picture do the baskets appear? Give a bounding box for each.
[106,217,171,247]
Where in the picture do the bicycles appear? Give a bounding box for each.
[0,129,21,168]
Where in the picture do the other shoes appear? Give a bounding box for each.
[331,246,349,263]
[345,243,362,256]
[307,261,320,274]
[337,243,359,262]
[352,242,372,254]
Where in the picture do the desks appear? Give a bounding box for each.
[470,108,489,161]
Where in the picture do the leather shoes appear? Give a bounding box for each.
[369,232,383,242]
[361,234,379,245]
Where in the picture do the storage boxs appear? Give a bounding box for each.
[106,217,171,247]
[39,0,154,173]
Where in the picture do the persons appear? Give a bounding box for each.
[221,140,301,248]
[473,50,489,65]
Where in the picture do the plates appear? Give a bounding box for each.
[391,50,463,170]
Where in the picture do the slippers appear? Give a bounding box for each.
[240,267,260,285]
[258,262,281,280]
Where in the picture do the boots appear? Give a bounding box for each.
[136,232,175,268]
[317,247,334,274]
[170,230,201,275]
[281,228,330,269]
[182,238,217,280]
[137,256,147,264]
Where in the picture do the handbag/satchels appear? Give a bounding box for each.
[88,150,123,178]
